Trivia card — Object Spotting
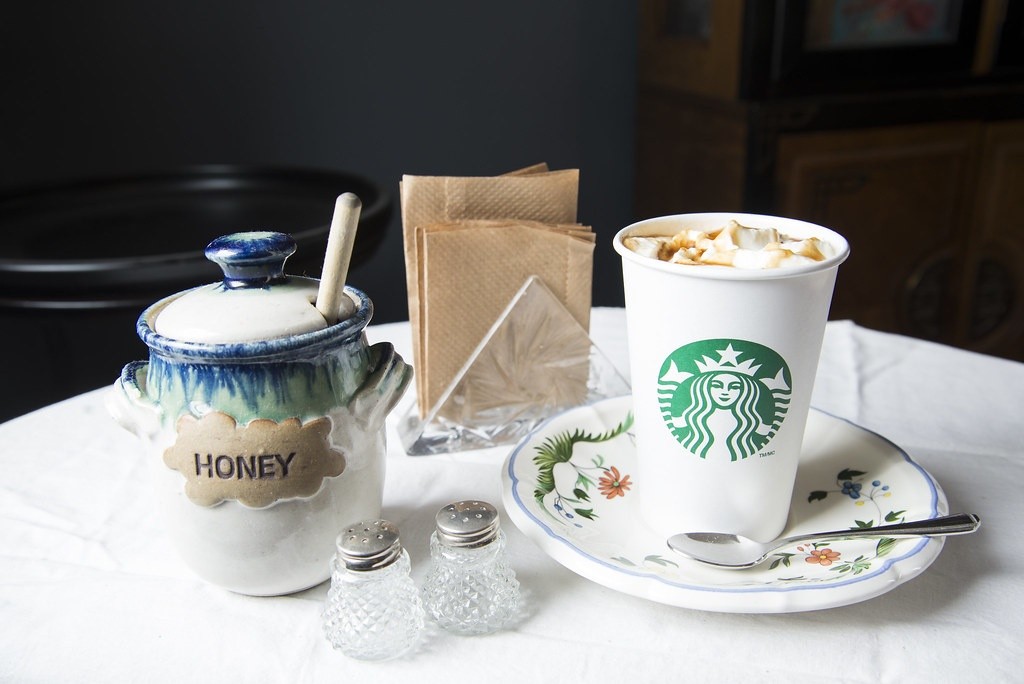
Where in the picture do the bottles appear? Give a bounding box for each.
[427,500,520,634]
[321,520,424,661]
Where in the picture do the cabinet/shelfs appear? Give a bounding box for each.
[634,0,1024,363]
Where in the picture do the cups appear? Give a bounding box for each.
[108,231,414,598]
[613,213,851,547]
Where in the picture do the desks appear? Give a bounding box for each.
[0,307,1024,682]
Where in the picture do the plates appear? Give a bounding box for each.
[501,394,950,614]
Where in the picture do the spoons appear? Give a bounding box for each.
[665,513,981,570]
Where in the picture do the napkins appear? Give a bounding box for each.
[398,162,597,421]
